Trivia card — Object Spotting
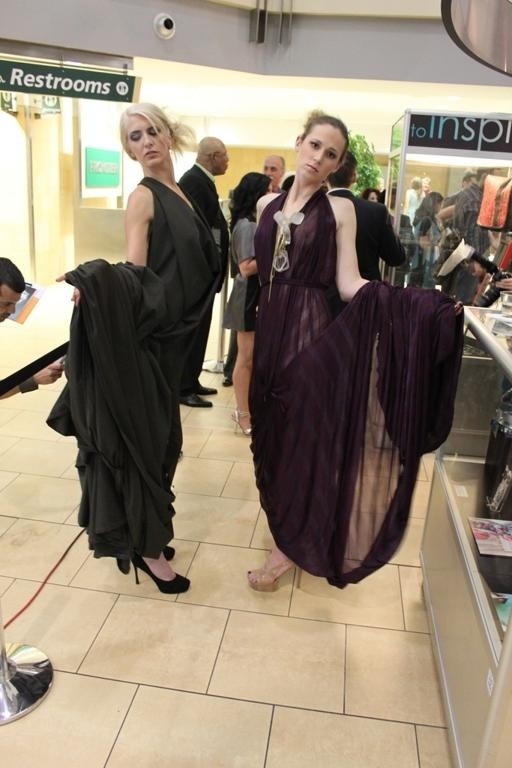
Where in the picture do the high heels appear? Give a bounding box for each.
[130,546,190,594]
[231,408,251,436]
[247,559,301,592]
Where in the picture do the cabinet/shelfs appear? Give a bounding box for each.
[414,306,511,767]
[375,104,510,458]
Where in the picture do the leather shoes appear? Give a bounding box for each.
[180,385,217,407]
[223,377,232,385]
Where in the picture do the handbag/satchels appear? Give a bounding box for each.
[476,173,512,230]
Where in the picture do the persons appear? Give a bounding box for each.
[1,257,67,400]
[45,103,223,596]
[177,137,227,408]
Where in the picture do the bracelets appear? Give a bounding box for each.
[20,376,37,392]
